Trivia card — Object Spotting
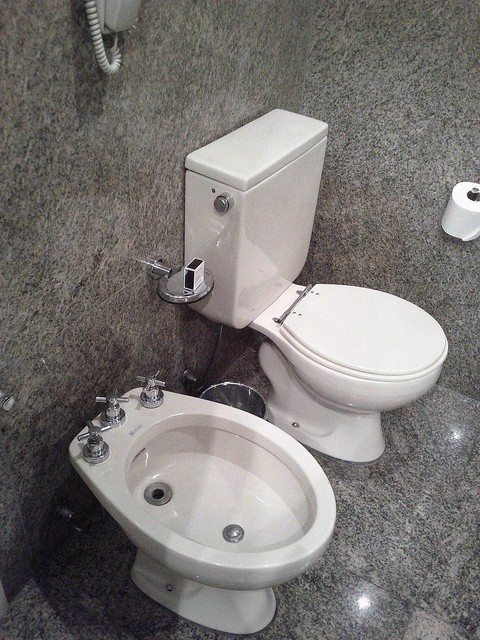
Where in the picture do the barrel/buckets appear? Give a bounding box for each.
[196,381,267,424]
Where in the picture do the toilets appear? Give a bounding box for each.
[68,370,337,636]
[184,109,448,464]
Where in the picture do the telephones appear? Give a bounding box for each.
[85,0,142,76]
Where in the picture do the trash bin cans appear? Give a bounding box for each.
[200,382,268,420]
